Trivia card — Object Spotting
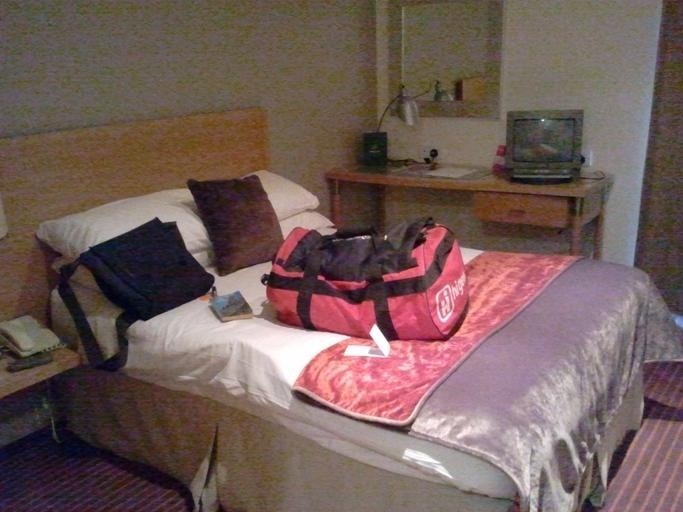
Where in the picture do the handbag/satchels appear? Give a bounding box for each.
[79,216,214,321]
[266,215,469,340]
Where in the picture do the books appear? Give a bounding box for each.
[211,290,255,322]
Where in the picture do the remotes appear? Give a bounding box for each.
[7,352,52,370]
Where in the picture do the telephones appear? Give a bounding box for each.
[0,315,61,357]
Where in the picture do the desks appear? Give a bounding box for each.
[325,157,615,260]
[2,342,83,450]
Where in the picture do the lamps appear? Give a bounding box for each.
[375,82,421,168]
[412,78,452,101]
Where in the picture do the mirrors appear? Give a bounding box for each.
[387,0,507,121]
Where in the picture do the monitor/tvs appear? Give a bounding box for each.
[505,108,584,184]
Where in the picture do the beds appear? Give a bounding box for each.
[1,106,683,511]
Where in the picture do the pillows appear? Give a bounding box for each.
[184,173,286,278]
[278,210,336,243]
[36,192,212,260]
[240,167,321,224]
[51,251,214,295]
